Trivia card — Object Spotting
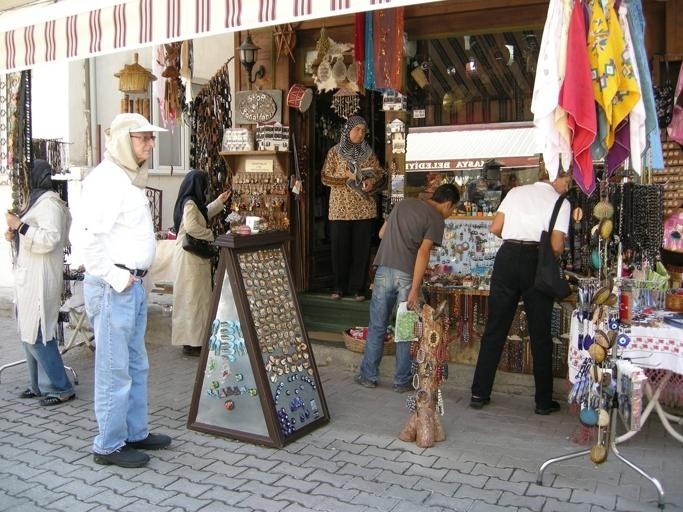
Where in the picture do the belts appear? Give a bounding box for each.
[116,264,148,278]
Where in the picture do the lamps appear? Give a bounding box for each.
[235,35,264,91]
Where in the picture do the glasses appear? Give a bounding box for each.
[131,135,156,142]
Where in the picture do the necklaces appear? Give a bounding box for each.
[428,296,571,375]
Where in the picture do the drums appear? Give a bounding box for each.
[286,83,313,113]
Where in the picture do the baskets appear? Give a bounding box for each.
[343,327,396,355]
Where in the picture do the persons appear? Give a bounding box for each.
[76,112,171,468]
[171,170,231,356]
[470,163,573,415]
[320,117,385,302]
[355,184,460,392]
[4,160,75,406]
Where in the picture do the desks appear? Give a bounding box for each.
[0,273,96,386]
[421,283,579,376]
[567,307,683,446]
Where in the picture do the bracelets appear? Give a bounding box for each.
[404,312,451,416]
[16,223,23,232]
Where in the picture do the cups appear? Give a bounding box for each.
[410,67,433,90]
[246,216,260,234]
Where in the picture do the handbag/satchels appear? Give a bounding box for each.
[534,231,572,301]
[183,234,213,258]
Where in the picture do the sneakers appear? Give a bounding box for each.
[331,293,339,301]
[356,296,364,302]
[535,401,561,415]
[40,393,76,405]
[393,384,414,393]
[470,395,490,408]
[126,433,171,451]
[22,389,35,398]
[355,374,376,388]
[183,346,201,356]
[93,447,149,467]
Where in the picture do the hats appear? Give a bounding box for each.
[129,113,169,132]
